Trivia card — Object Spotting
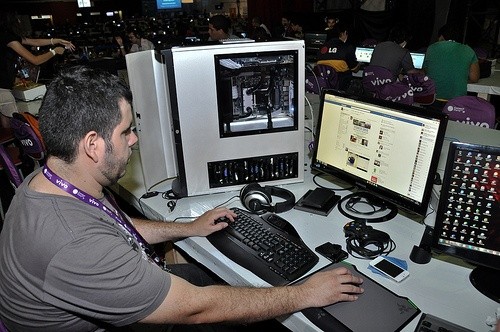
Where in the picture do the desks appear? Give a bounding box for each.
[104,131,499,332]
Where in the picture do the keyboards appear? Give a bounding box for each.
[206,207,319,286]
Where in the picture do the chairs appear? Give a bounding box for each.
[304,33,500,130]
[0,112,47,225]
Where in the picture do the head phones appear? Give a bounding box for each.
[239,183,295,213]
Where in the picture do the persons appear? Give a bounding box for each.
[369,29,420,82]
[0,64,363,332]
[251,17,273,41]
[314,21,364,95]
[419,23,481,111]
[279,14,295,40]
[208,15,239,41]
[116,27,155,56]
[324,15,341,38]
[0,1,78,122]
[289,20,306,42]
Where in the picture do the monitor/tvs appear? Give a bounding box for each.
[354,46,374,63]
[310,88,449,217]
[410,52,425,70]
[431,142,500,271]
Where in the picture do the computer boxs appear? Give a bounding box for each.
[124,37,304,197]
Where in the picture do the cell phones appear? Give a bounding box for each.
[370,256,409,283]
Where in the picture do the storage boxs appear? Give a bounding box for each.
[0,79,48,116]
